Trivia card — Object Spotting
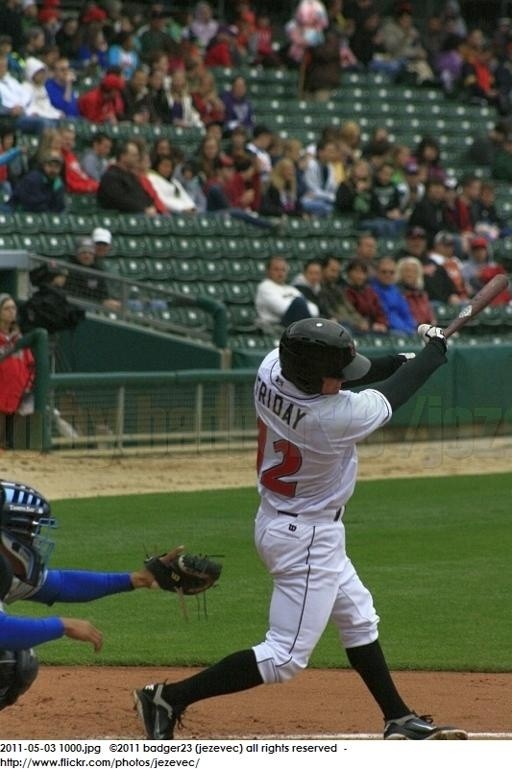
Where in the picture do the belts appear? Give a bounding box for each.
[278,508,341,521]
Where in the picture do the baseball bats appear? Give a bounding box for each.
[444,273,509,334]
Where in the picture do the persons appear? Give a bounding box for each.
[0,482,223,710]
[132,320,467,739]
[2,2,509,449]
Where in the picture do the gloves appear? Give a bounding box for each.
[418,324,447,343]
[398,352,416,360]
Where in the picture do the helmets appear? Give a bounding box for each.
[280,318,372,394]
[0,478,59,589]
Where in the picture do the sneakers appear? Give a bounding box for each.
[384,709,469,740]
[132,683,187,740]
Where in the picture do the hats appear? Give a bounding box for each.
[92,228,112,245]
[405,226,488,249]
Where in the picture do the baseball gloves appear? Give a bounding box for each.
[144,548,224,595]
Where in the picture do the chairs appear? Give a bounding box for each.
[0,54,512,354]
